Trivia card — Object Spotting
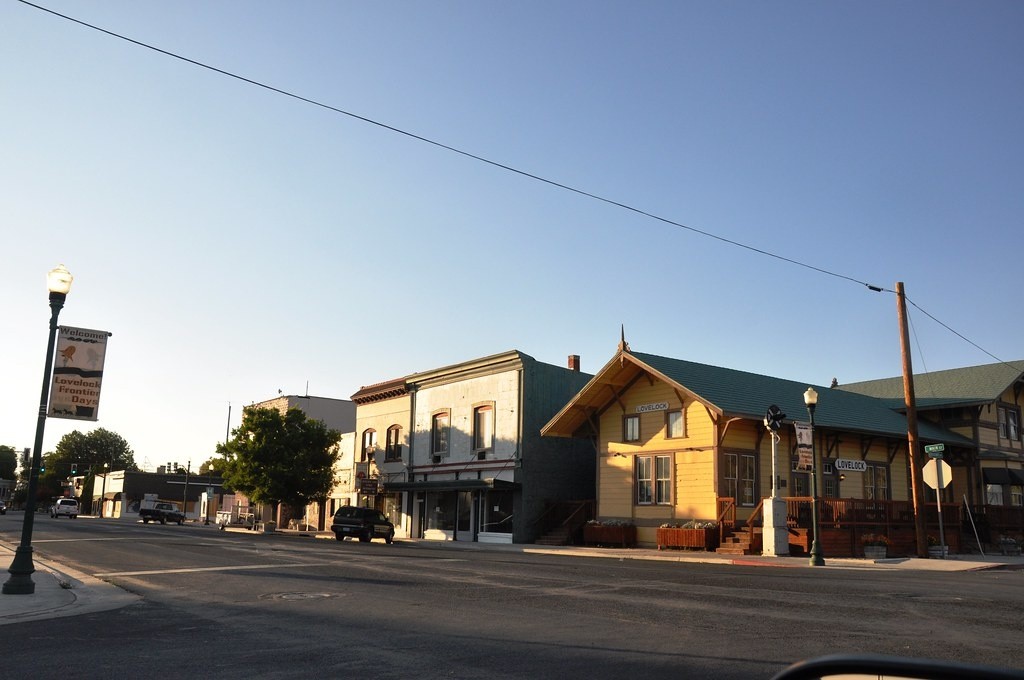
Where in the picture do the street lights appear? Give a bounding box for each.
[362,444,374,506]
[203,463,215,526]
[99,462,108,519]
[803,386,825,567]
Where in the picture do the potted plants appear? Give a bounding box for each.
[263,519,276,532]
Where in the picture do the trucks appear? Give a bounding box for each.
[140,502,187,525]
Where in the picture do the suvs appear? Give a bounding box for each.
[331,505,395,544]
[50,498,79,519]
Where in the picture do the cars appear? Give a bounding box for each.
[0,501,7,515]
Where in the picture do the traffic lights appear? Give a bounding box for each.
[40,460,45,472]
[71,464,78,476]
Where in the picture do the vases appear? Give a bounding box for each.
[1001,544,1022,556]
[583,525,636,549]
[928,545,948,558]
[864,545,888,560]
[656,528,717,551]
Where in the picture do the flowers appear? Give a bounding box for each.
[860,533,894,547]
[999,536,1017,545]
[660,521,720,528]
[926,535,940,546]
[587,518,634,526]
